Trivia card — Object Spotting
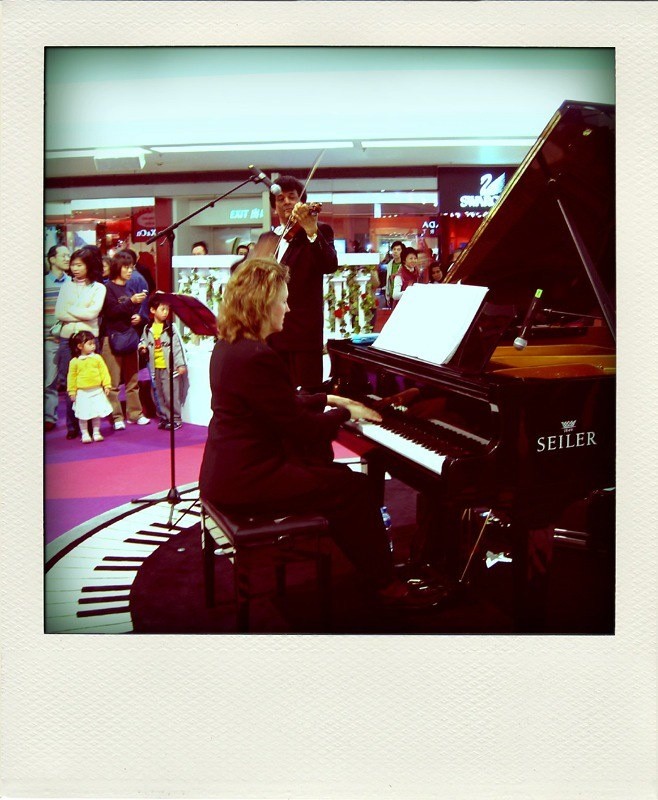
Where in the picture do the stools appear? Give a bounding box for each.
[199,495,338,635]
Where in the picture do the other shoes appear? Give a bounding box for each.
[131,414,150,425]
[82,433,92,443]
[114,418,125,430]
[374,577,447,612]
[45,421,56,431]
[158,418,182,430]
[66,430,78,440]
[93,431,103,442]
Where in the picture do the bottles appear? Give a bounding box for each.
[380,507,394,552]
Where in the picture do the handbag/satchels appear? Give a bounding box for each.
[109,327,140,354]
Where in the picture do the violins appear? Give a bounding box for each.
[230,202,324,277]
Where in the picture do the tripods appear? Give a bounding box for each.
[130,177,256,527]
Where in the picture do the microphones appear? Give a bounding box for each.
[249,164,281,195]
[515,289,543,351]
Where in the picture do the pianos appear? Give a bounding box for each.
[295,98,615,633]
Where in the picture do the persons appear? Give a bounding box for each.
[43,176,445,442]
[200,259,449,607]
